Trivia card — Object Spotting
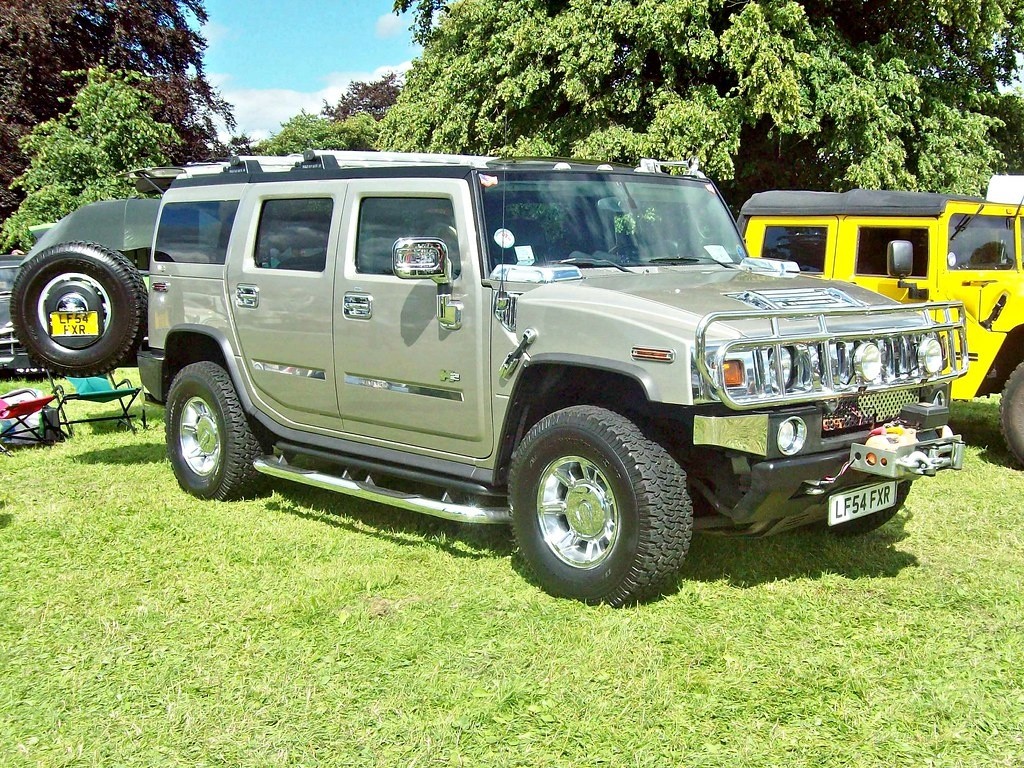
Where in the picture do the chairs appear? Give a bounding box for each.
[45,367,143,439]
[0,388,66,457]
[253,210,549,276]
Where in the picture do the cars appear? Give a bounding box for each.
[0,250,83,375]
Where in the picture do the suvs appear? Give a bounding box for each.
[731,186,1024,475]
[7,151,973,610]
